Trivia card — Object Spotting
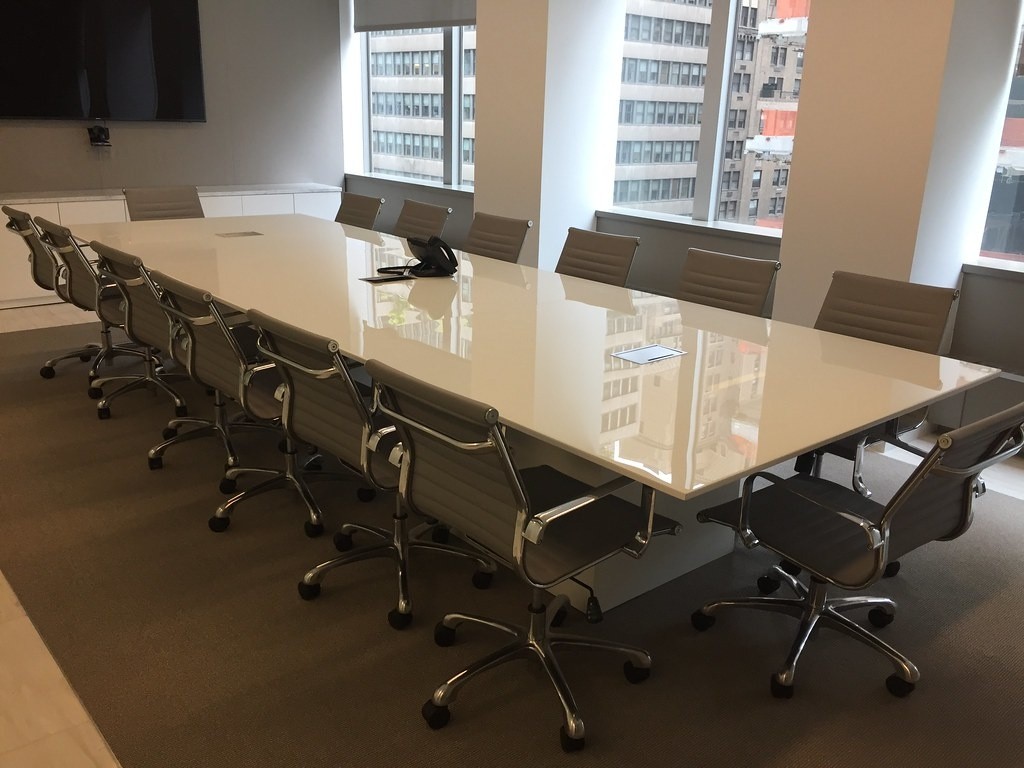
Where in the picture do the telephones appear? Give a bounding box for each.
[406,233,459,278]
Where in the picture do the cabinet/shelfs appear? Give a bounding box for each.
[1,183,342,310]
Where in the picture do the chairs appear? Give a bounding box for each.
[90,241,296,472]
[673,246,782,316]
[792,271,962,479]
[149,270,377,539]
[1,206,164,384]
[462,212,534,264]
[688,402,1024,699]
[35,216,218,419]
[363,358,682,751]
[553,227,642,288]
[391,197,452,243]
[247,309,497,632]
[335,192,386,231]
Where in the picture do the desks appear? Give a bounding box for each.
[61,213,1008,499]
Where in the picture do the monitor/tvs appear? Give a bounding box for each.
[0,0,207,122]
